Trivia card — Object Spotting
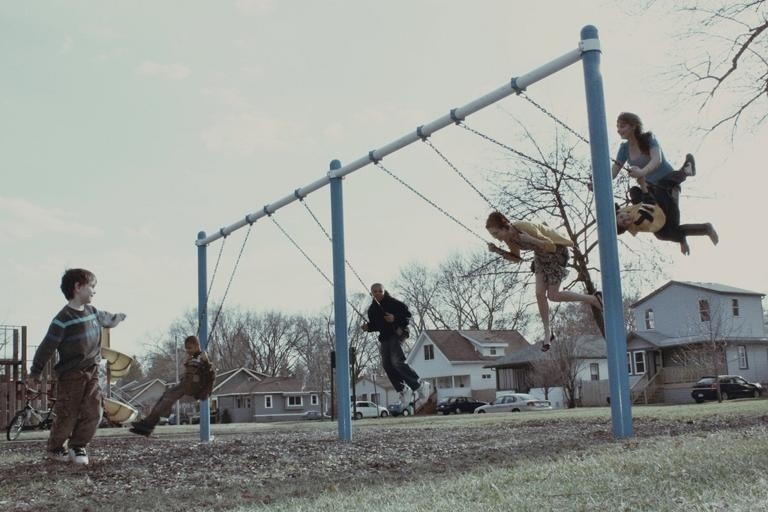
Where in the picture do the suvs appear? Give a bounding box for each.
[690,374,763,403]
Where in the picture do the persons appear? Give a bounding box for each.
[587,113,719,256]
[617,153,696,233]
[486,211,602,352]
[129,335,216,436]
[29,268,125,465]
[359,284,430,409]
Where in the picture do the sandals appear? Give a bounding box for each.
[540,333,557,352]
[594,290,604,312]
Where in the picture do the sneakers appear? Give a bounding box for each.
[46,446,69,462]
[418,381,432,402]
[129,419,154,438]
[68,440,89,465]
[400,384,411,404]
[681,152,696,177]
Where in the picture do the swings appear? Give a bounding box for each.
[456,91,679,204]
[376,138,568,272]
[192,223,252,400]
[268,198,409,340]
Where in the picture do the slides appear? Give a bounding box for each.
[99,347,139,424]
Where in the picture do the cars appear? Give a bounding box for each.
[191,411,214,424]
[389,400,414,417]
[302,410,326,417]
[472,392,553,414]
[350,400,390,421]
[159,417,169,425]
[436,396,489,415]
[167,412,189,425]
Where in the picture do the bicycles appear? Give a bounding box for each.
[3,380,56,444]
